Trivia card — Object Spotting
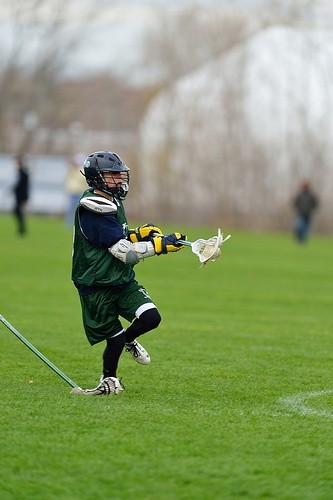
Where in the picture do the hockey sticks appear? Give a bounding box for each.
[0,314,120,395]
[153,228,232,269]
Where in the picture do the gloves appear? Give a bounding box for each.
[127,224,184,246]
[149,233,196,258]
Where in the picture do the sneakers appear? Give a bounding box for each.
[100,375,126,394]
[125,340,151,365]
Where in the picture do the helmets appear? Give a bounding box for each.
[83,151,129,188]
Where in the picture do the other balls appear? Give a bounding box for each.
[209,247,221,258]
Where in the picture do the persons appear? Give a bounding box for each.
[12,154,30,237]
[293,181,318,243]
[71,151,188,394]
[63,154,92,227]
